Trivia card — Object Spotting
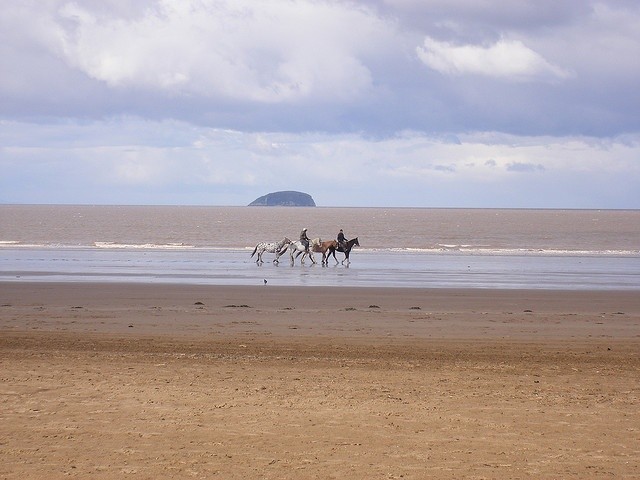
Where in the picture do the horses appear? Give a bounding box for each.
[321,237,360,264]
[250,237,293,263]
[295,239,338,264]
[279,238,323,264]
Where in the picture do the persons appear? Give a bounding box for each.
[300,228,310,251]
[337,229,348,253]
